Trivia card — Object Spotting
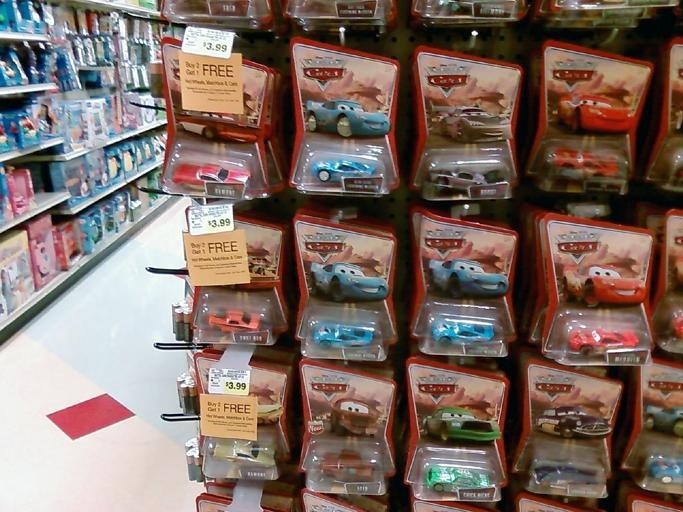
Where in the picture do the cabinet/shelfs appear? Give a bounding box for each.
[1,0,181,346]
